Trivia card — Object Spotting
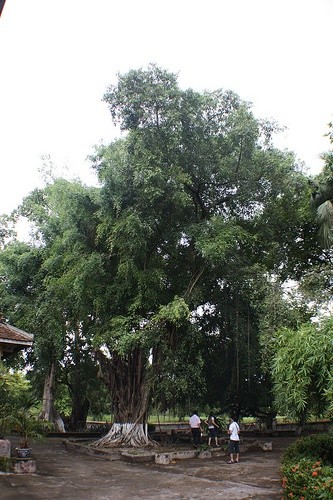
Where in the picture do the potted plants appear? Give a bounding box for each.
[0,407,55,457]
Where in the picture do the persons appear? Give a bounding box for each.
[225,416,241,465]
[189,409,204,448]
[205,410,221,447]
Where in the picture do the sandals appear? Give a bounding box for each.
[227,460,239,464]
